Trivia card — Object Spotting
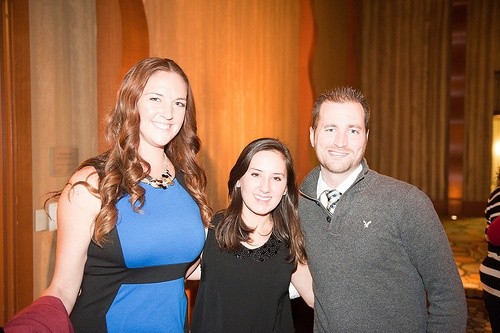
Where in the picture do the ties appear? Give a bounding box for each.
[325,190,340,215]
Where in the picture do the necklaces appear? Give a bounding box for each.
[144,153,175,190]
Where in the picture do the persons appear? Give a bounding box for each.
[184,137,315,333]
[0,56,215,333]
[289,85,468,333]
